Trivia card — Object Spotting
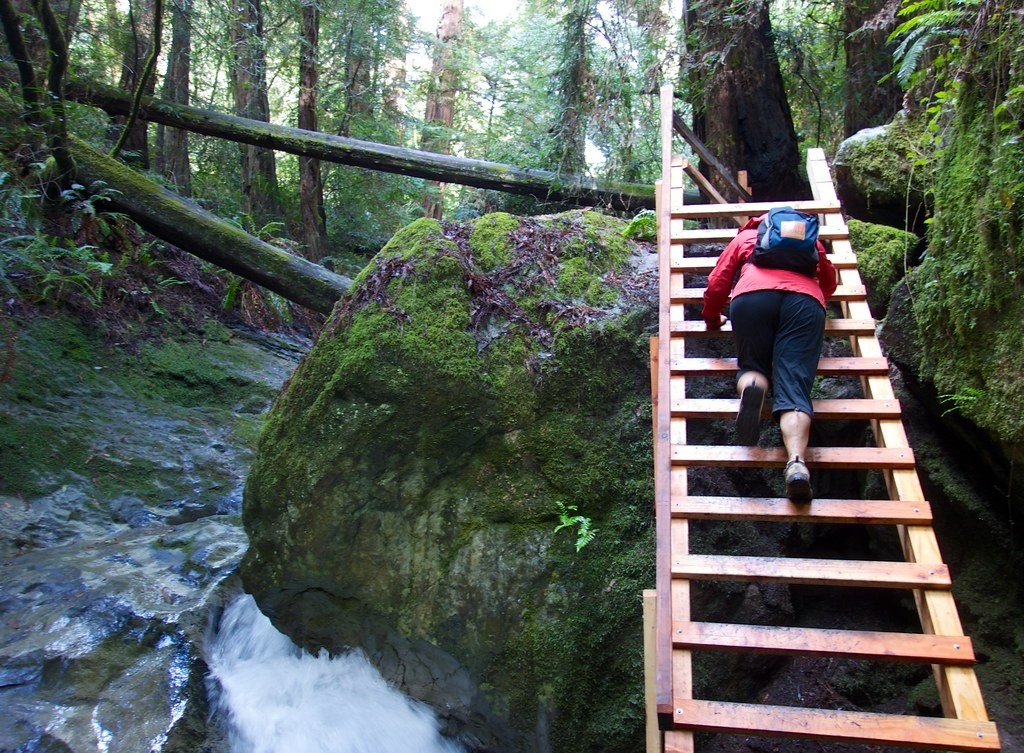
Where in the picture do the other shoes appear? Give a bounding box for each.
[732,382,766,447]
[782,455,814,501]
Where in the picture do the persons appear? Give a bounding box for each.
[702,213,840,504]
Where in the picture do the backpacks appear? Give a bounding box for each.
[736,206,821,277]
